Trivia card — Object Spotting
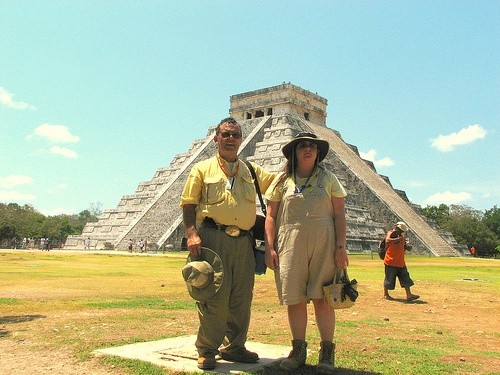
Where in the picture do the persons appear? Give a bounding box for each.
[12,233,53,251]
[83,238,87,250]
[471,245,476,257]
[88,237,93,250]
[383,222,420,301]
[126,238,148,254]
[180,117,280,369]
[263,131,349,373]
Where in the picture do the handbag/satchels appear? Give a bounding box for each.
[250,214,266,254]
[324,264,357,310]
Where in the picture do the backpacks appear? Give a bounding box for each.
[378,238,386,260]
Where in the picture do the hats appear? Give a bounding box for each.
[183,247,223,302]
[281,132,329,162]
[395,221,406,232]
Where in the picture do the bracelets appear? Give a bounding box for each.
[337,246,347,249]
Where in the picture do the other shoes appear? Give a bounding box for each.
[407,292,420,301]
[384,294,393,299]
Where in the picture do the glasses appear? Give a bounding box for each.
[219,131,243,140]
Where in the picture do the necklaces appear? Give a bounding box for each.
[294,166,316,194]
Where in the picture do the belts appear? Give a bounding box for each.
[202,217,247,237]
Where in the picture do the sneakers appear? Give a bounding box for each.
[280,338,308,370]
[318,341,337,373]
[198,356,216,369]
[219,347,259,362]
